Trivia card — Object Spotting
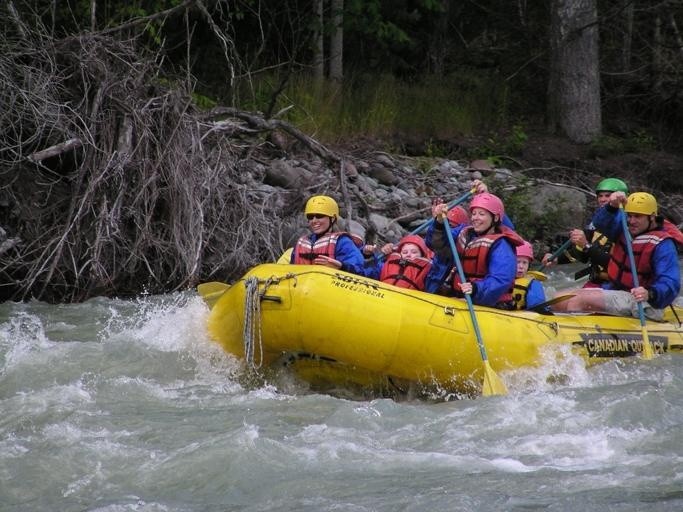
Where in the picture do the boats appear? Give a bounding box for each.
[205,245,682,404]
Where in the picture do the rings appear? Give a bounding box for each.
[639,294,642,298]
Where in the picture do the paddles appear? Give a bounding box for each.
[527,293,576,312]
[197,282,232,310]
[441,212,505,395]
[618,202,655,359]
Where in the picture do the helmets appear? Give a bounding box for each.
[396,235,427,257]
[445,204,468,225]
[623,190,658,216]
[595,178,628,193]
[468,192,506,222]
[305,195,340,223]
[516,240,534,260]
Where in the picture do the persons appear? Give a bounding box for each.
[290,179,552,318]
[542,177,628,290]
[545,190,683,322]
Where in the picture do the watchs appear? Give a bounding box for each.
[582,242,591,251]
